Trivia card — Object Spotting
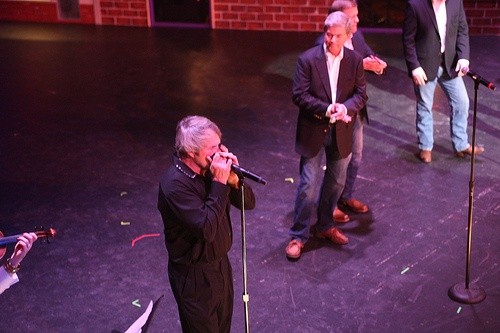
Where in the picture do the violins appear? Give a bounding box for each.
[0,227,55,259]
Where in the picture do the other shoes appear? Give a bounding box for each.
[316,227,348,244]
[456,145,485,157]
[338,198,368,213]
[285,239,304,258]
[418,150,432,163]
[333,208,349,222]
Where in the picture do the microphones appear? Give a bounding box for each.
[209,153,267,185]
[461,66,496,91]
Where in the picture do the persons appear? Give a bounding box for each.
[156,114,255,333]
[284,9,368,258]
[0,232,38,295]
[402,0,484,162]
[315,0,387,220]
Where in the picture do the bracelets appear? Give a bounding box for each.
[3,258,21,273]
